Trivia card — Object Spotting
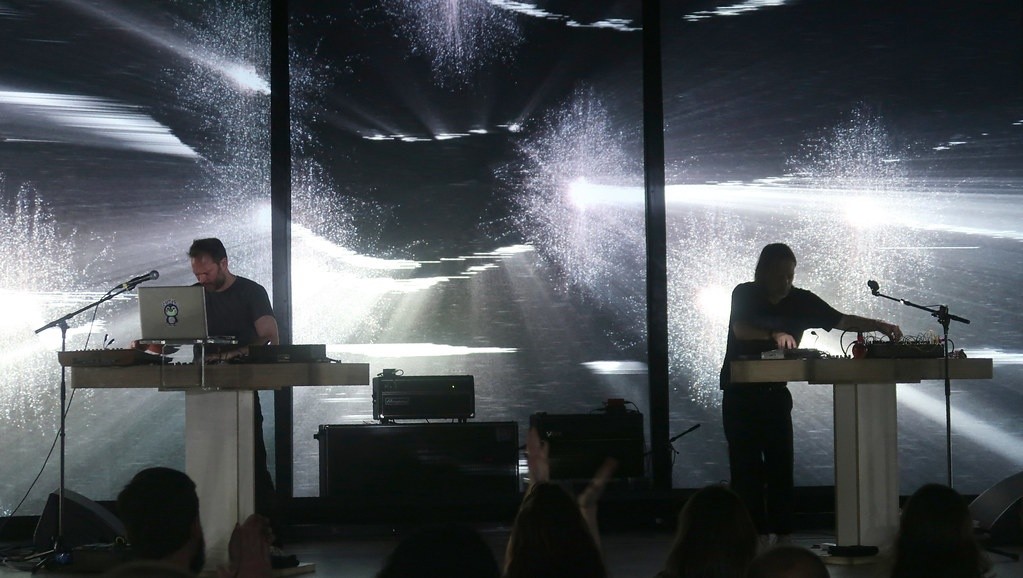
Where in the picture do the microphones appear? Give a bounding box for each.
[116,271,159,289]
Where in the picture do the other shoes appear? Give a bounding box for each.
[756,533,778,553]
[776,533,794,544]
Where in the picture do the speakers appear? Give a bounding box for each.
[31,487,129,552]
[968,470,1023,547]
[317,409,644,526]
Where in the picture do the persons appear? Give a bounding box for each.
[504,429,615,578]
[380,526,501,578]
[720,242,903,544]
[656,485,759,578]
[116,467,276,578]
[891,483,989,578]
[747,545,829,578]
[131,238,281,549]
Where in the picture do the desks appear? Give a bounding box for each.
[730,357,993,565]
[71,363,369,576]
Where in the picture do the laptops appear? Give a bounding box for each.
[137,285,236,340]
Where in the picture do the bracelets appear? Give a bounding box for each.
[768,328,774,340]
